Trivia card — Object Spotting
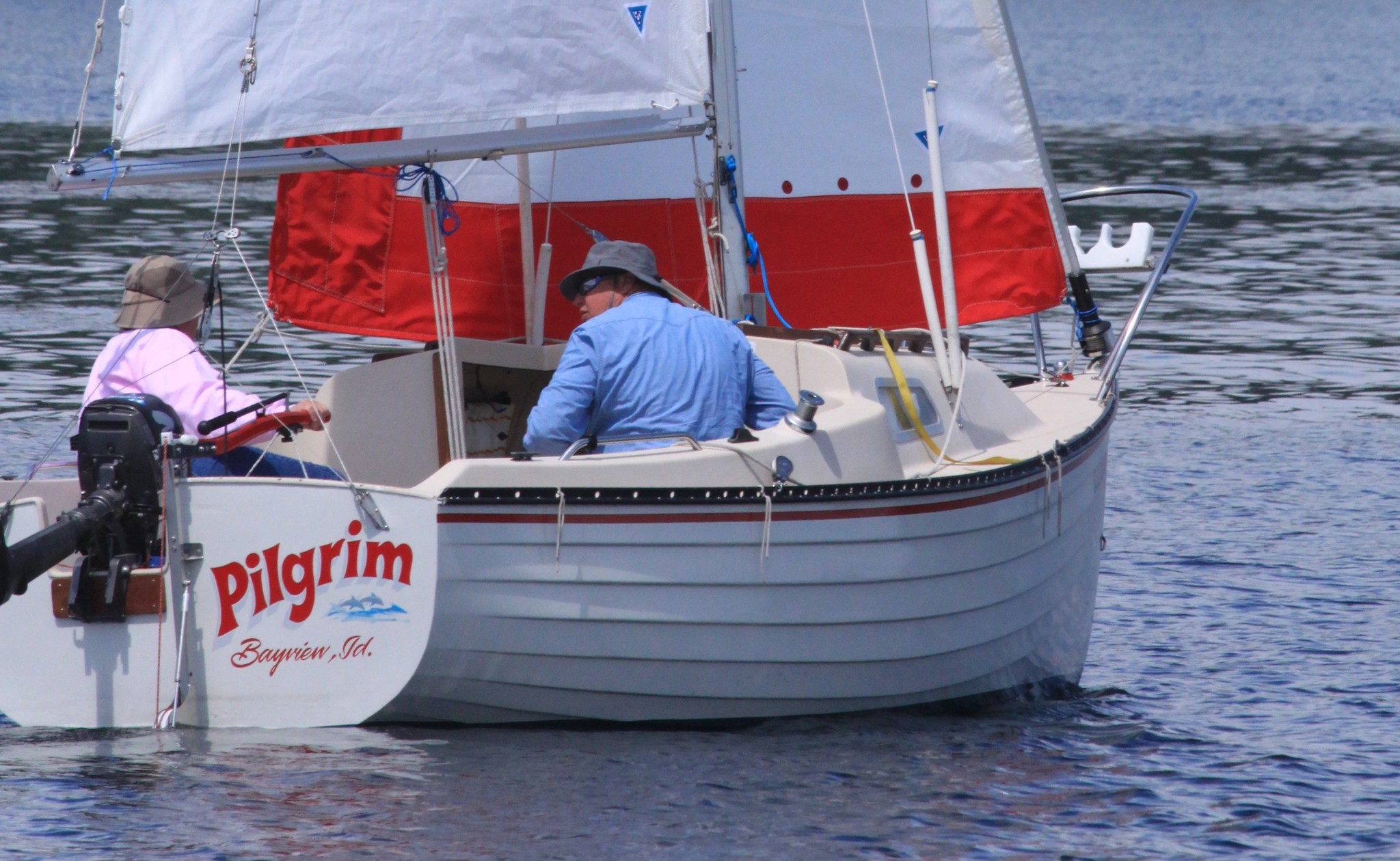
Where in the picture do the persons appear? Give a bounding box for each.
[78,256,349,478]
[522,239,800,454]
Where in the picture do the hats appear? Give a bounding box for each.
[560,240,675,302]
[113,254,225,329]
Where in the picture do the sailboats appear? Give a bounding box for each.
[0,0,1203,729]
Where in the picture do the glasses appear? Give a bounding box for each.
[578,270,627,295]
